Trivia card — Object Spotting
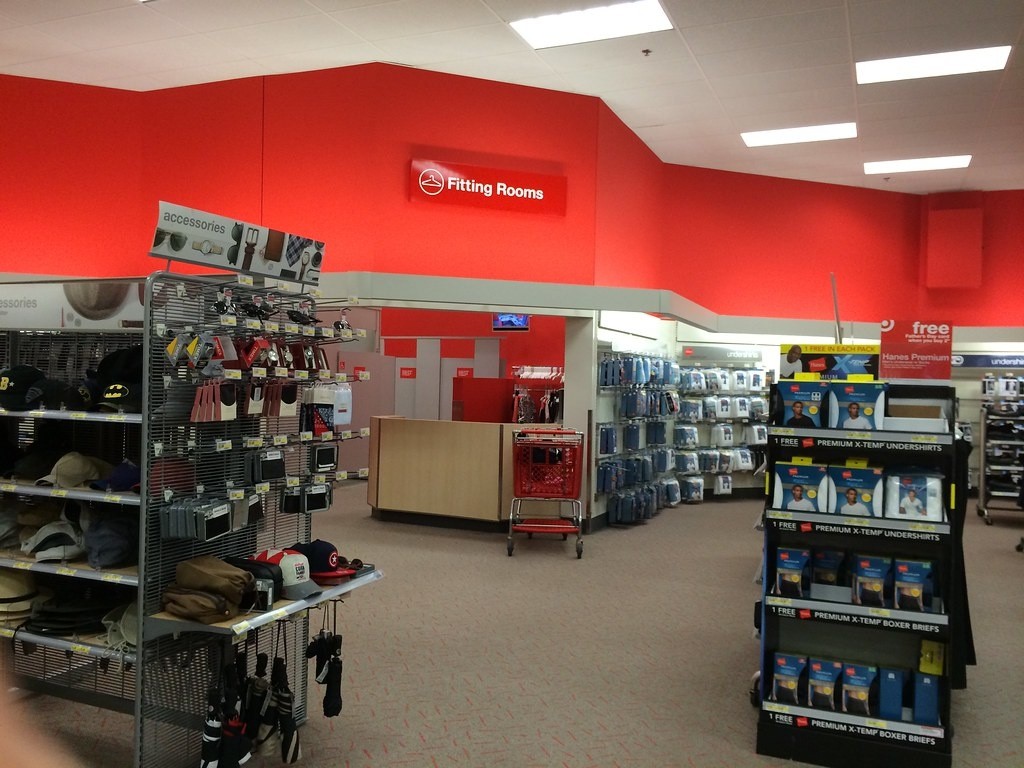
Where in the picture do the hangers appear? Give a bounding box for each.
[512,364,565,383]
[514,387,558,401]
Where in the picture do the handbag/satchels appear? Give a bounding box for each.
[161,549,287,625]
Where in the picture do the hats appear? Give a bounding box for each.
[0,567,140,673]
[0,346,146,416]
[0,500,141,571]
[248,539,356,601]
[0,435,140,494]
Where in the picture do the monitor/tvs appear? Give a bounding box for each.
[492,312,530,332]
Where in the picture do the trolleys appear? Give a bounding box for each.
[506,428,588,559]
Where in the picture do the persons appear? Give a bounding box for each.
[780,345,802,377]
[774,674,798,704]
[809,679,835,710]
[788,485,816,511]
[900,490,926,515]
[856,576,886,607]
[840,489,870,516]
[777,568,803,598]
[895,581,924,612]
[844,403,872,429]
[843,684,869,715]
[786,402,817,429]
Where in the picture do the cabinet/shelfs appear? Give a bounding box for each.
[0,270,384,768]
[748,351,1024,768]
